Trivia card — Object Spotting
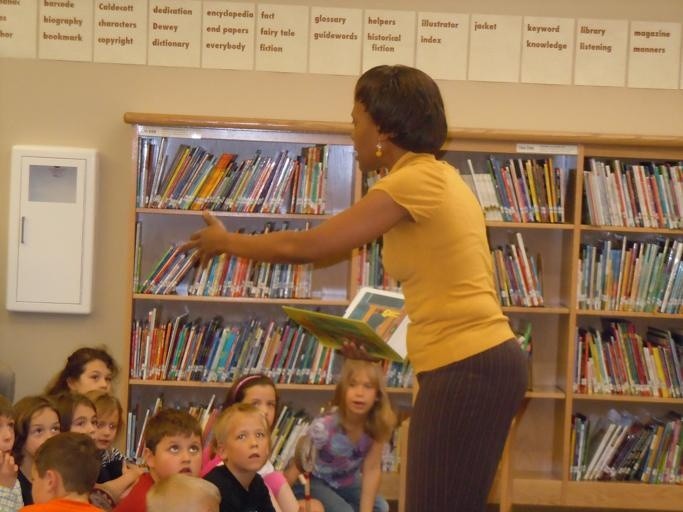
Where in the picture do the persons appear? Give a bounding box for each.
[1,347,401,510]
[176,65,531,512]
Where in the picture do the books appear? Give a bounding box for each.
[581,156,683,228]
[269,403,337,469]
[460,156,565,223]
[132,220,314,298]
[511,322,532,352]
[136,136,328,215]
[578,234,683,313]
[127,394,221,462]
[571,409,683,484]
[575,318,683,399]
[130,306,413,388]
[282,288,411,365]
[357,236,401,291]
[489,231,543,307]
